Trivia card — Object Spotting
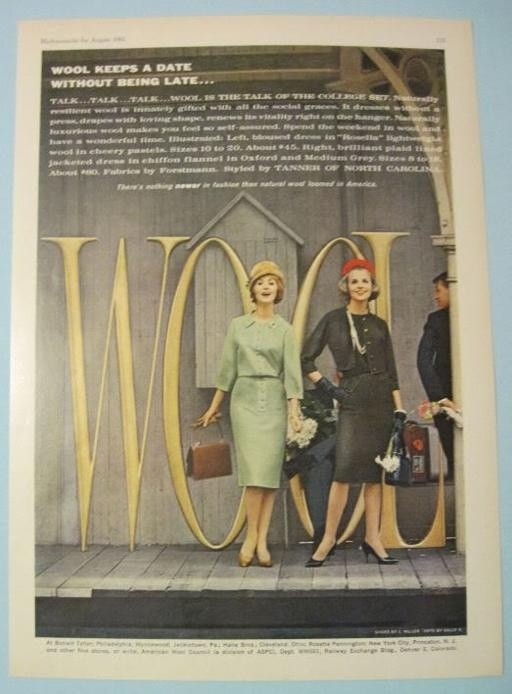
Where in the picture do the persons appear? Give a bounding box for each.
[416,270,455,471]
[195,259,304,569]
[300,258,406,568]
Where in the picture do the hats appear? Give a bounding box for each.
[247,260,285,291]
[341,259,373,277]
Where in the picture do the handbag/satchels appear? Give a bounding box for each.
[186,423,231,479]
[379,435,413,487]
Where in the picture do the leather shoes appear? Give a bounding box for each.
[238,546,273,568]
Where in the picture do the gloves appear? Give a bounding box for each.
[317,379,351,404]
[391,411,407,435]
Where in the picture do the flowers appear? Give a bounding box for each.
[375,397,463,473]
[286,416,318,449]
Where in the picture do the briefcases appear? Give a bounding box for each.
[402,420,430,484]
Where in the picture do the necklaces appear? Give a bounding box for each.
[347,308,368,354]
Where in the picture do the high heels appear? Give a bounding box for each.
[361,540,398,565]
[305,542,337,567]
